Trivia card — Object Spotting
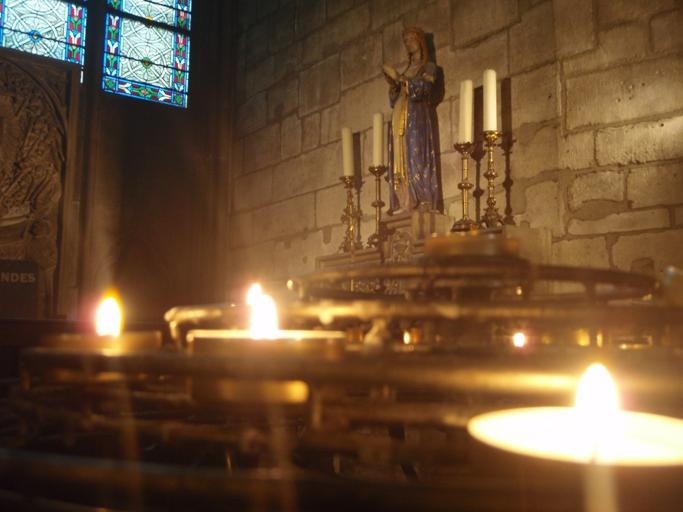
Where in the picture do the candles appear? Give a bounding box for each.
[339,111,385,177]
[458,66,499,144]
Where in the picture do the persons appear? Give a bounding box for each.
[380,25,441,215]
